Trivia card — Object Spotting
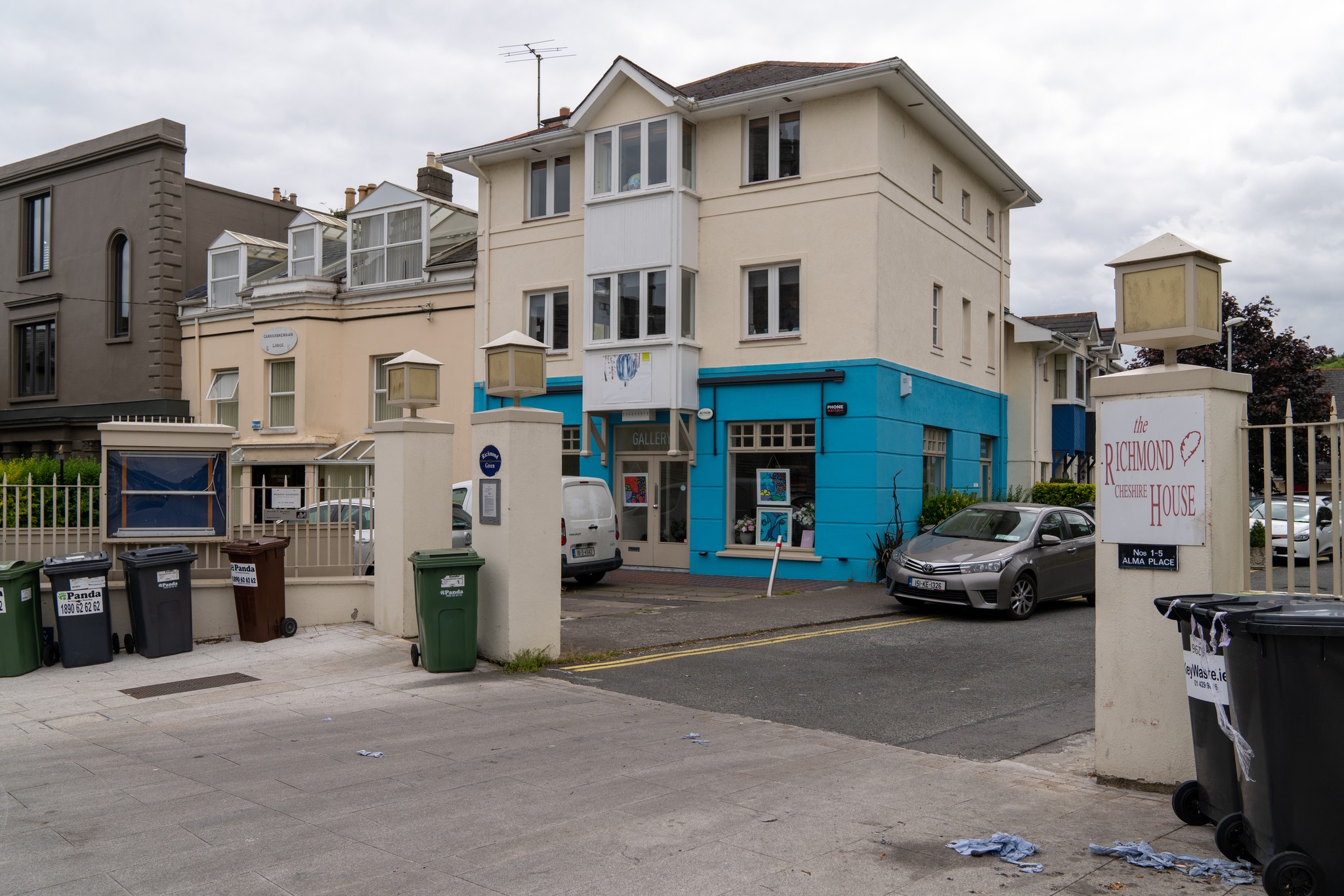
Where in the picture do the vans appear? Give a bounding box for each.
[452,475,623,586]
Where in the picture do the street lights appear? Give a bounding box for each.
[1221,316,1247,373]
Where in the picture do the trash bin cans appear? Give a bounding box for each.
[1155,592,1344,896]
[117,543,198,658]
[220,535,297,644]
[42,551,120,668]
[408,548,485,673]
[0,559,56,678]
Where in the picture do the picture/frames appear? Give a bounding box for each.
[757,469,791,506]
[756,507,793,546]
[623,473,648,506]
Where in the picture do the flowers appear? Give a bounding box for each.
[792,502,814,527]
[731,515,756,532]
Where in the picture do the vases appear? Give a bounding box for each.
[739,532,755,544]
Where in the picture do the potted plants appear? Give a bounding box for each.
[1249,520,1276,570]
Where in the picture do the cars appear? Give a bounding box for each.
[1249,495,1342,563]
[276,496,474,576]
[884,500,1096,621]
